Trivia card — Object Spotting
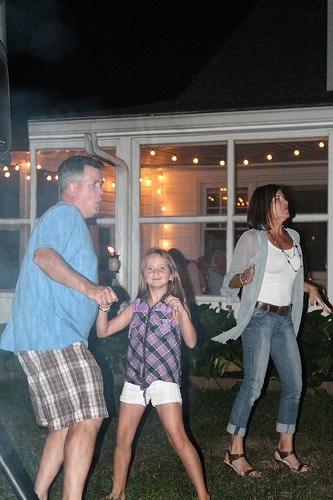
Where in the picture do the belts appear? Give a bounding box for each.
[255,301,292,315]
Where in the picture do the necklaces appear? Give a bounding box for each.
[267,228,302,272]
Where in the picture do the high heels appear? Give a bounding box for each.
[224,449,257,478]
[274,449,308,473]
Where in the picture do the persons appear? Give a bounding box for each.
[210,184,332,477]
[0,155,118,500]
[95,246,211,500]
[168,248,226,296]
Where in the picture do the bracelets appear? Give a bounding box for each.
[239,274,244,284]
[97,305,110,312]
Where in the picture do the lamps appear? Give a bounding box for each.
[107,254,120,286]
